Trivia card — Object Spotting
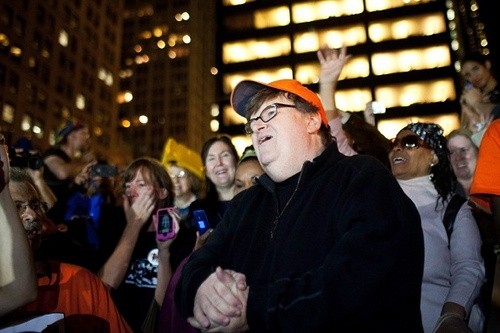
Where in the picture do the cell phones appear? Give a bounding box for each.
[193,210,210,236]
[156,207,176,241]
[91,165,116,177]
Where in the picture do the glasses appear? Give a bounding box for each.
[384,135,434,152]
[244,103,296,133]
[169,170,186,178]
[15,202,48,217]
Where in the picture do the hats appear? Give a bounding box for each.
[230,79,328,128]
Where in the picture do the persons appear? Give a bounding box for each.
[235,145,265,193]
[315,41,485,333]
[173,79,425,333]
[469,119,500,242]
[447,128,479,198]
[98,159,182,333]
[7,167,134,333]
[459,51,500,131]
[336,108,393,160]
[183,136,240,247]
[166,160,198,218]
[42,121,96,207]
